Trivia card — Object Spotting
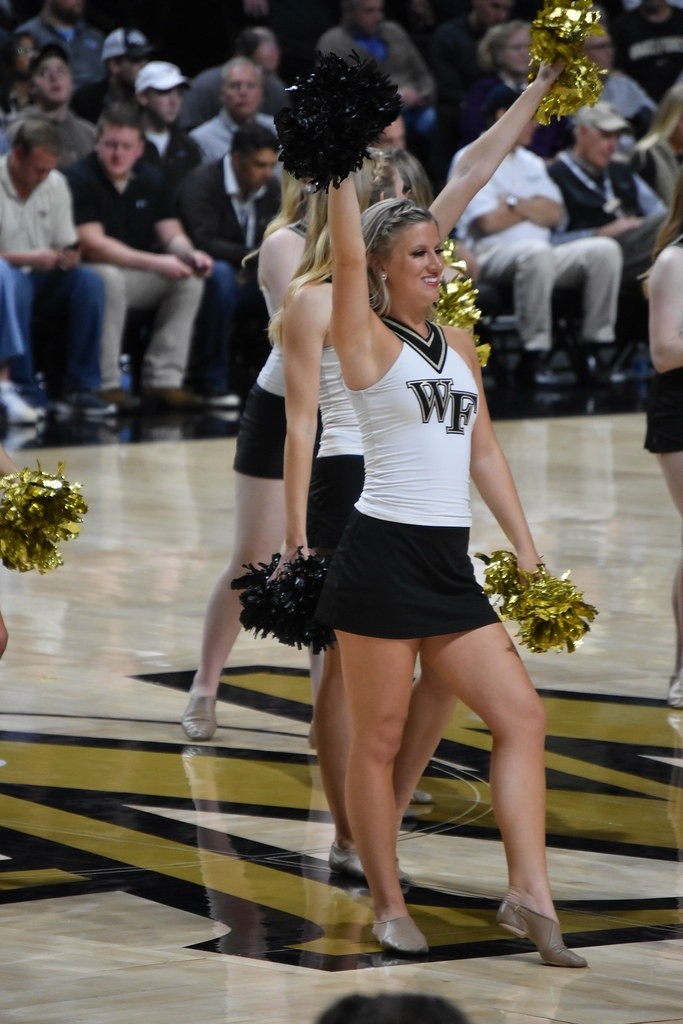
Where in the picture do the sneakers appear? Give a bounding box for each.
[12,377,59,419]
[62,391,116,417]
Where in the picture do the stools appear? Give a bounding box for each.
[473,285,621,404]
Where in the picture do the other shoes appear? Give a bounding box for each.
[371,916,430,956]
[0,379,39,427]
[138,386,203,409]
[565,338,626,389]
[409,789,432,805]
[666,675,683,709]
[307,719,317,749]
[100,387,141,412]
[513,350,564,391]
[205,392,242,408]
[494,901,588,969]
[181,686,217,741]
[328,840,412,884]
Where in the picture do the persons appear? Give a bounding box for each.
[325,176,591,970]
[180,53,567,886]
[0,439,85,659]
[63,102,216,415]
[643,162,683,707]
[444,0,683,386]
[0,0,437,174]
[189,123,280,409]
[0,121,116,427]
[45,174,47,177]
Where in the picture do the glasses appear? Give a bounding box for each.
[124,53,154,62]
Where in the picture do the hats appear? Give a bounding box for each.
[133,61,191,96]
[576,99,633,138]
[100,25,155,64]
[27,39,72,77]
